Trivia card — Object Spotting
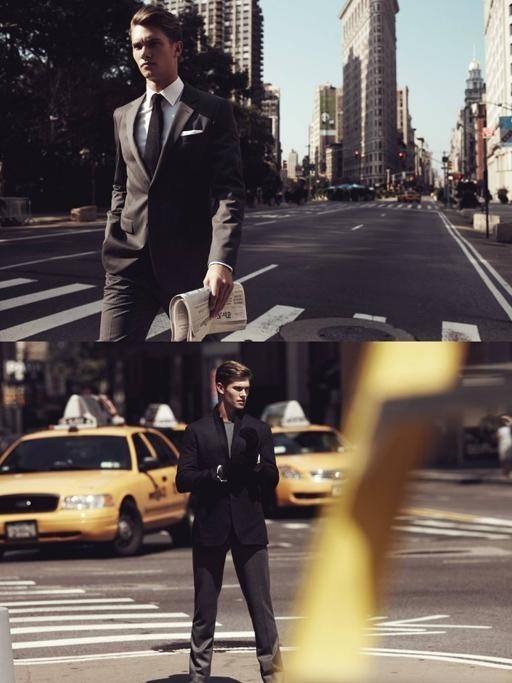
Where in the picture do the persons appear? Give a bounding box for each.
[174,357,284,682]
[94,4,249,344]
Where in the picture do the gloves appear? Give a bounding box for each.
[238,426,261,459]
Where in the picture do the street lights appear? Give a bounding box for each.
[466,100,491,239]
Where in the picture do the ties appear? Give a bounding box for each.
[142,93,163,180]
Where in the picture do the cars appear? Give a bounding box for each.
[398,189,421,203]
[253,399,358,518]
[0,391,195,557]
